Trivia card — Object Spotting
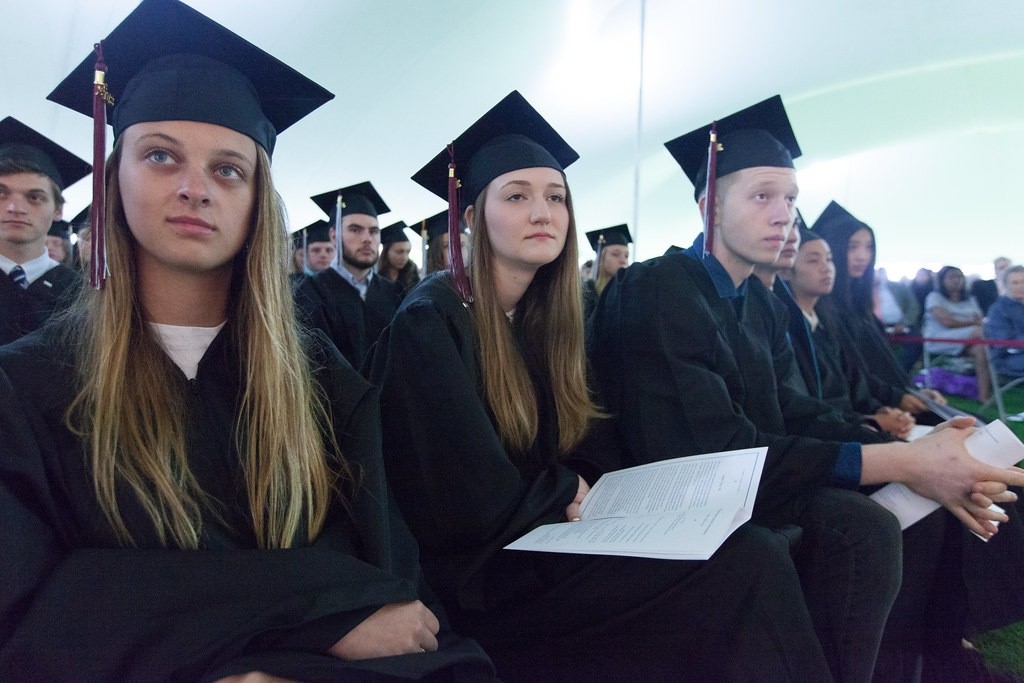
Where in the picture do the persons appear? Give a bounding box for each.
[0,116,94,345]
[377,220,421,293]
[585,92,1024,682]
[408,206,471,273]
[985,266,1024,374]
[286,219,336,294]
[580,223,634,318]
[2,1,504,683]
[921,267,990,404]
[371,89,834,683]
[295,182,405,375]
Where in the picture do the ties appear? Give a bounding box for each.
[9,266,27,293]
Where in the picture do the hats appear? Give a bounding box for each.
[0,116,93,192]
[46,0,336,290]
[410,205,470,281]
[70,205,93,233]
[48,220,72,239]
[311,181,392,264]
[794,206,820,243]
[664,95,803,259]
[377,219,408,242]
[411,89,579,301]
[290,219,331,274]
[811,200,857,238]
[586,224,634,280]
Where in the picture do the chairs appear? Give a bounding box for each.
[919,324,1024,425]
